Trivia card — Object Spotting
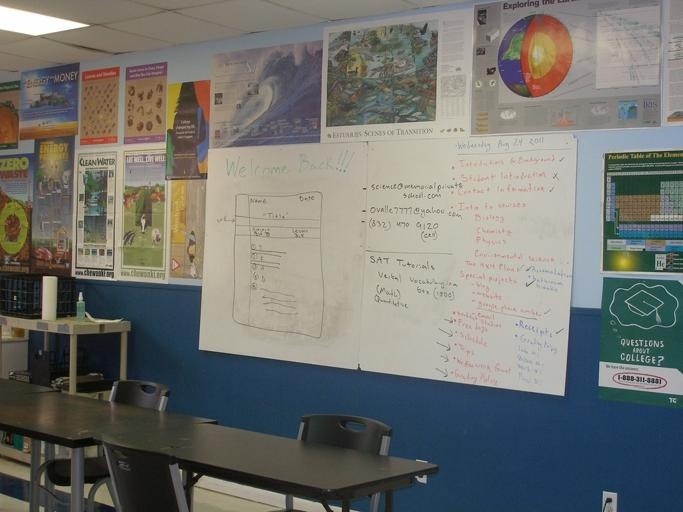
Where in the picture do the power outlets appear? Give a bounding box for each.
[601,491,618,512]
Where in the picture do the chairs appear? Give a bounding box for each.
[103,445,201,511]
[285,414,393,512]
[35,379,170,511]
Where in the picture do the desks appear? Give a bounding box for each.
[93,402,440,511]
[2,379,109,511]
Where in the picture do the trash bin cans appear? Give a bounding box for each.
[49,375,115,459]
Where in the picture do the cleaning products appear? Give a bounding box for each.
[76,292,85,319]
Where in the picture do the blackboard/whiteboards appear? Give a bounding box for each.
[197,132,579,397]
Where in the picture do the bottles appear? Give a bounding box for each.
[76,291,87,321]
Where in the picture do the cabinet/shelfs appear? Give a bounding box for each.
[0,315,132,469]
